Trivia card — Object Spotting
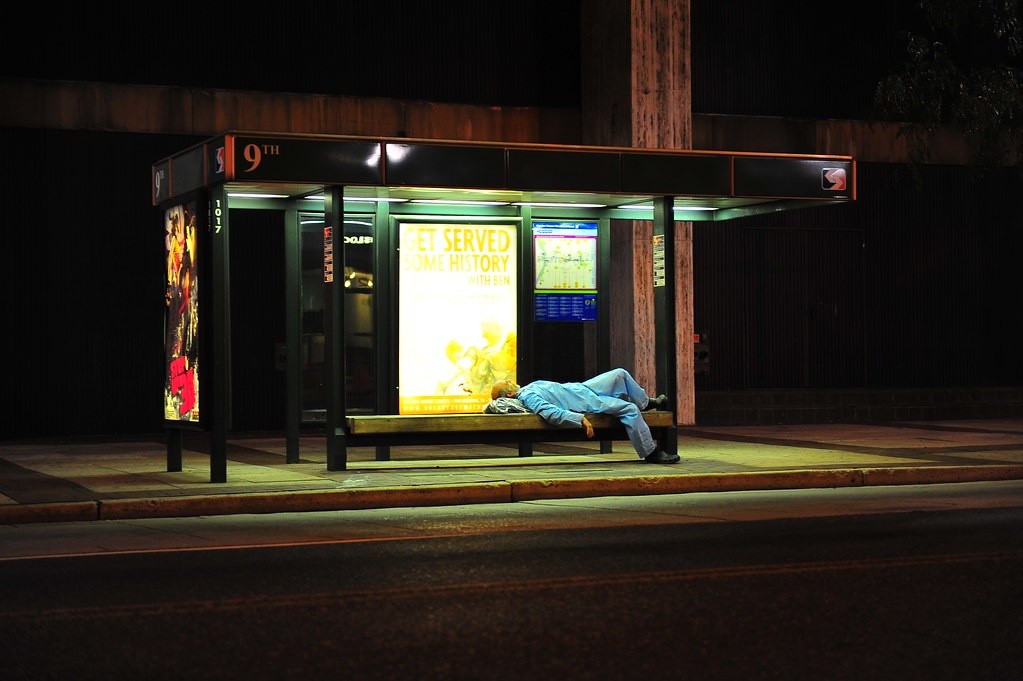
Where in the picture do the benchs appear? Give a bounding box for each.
[343,407,674,461]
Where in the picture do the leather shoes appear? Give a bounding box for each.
[645,447,680,463]
[642,394,668,411]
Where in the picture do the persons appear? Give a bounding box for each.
[491,367,680,463]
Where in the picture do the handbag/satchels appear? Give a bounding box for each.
[483,396,530,413]
